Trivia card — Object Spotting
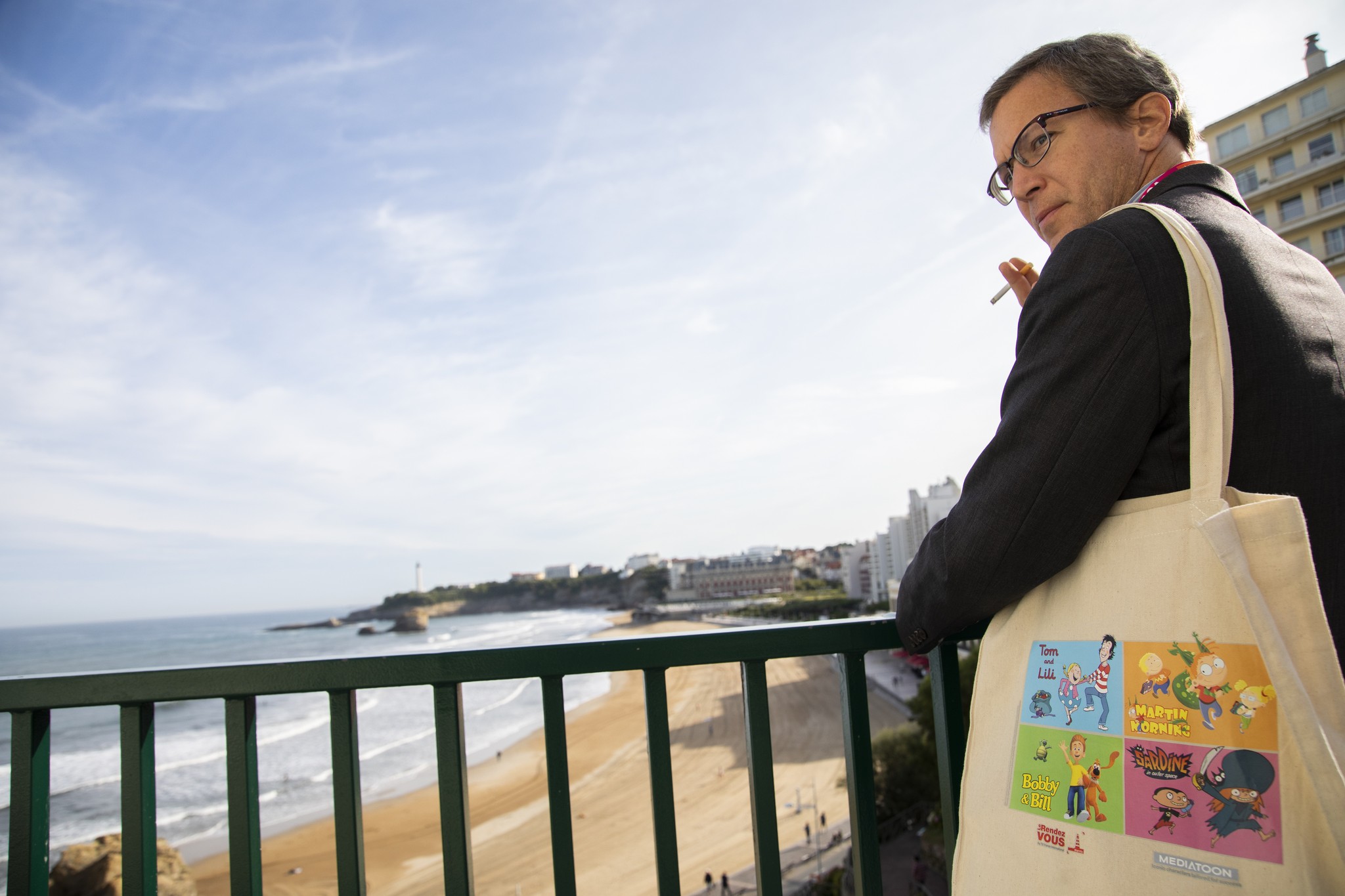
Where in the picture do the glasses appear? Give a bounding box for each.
[986,101,1105,206]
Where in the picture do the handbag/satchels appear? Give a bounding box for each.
[951,202,1345,895]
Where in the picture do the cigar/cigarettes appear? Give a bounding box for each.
[990,263,1033,305]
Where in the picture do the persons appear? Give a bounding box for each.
[890,31,1345,673]
[804,812,829,845]
[705,871,733,896]
